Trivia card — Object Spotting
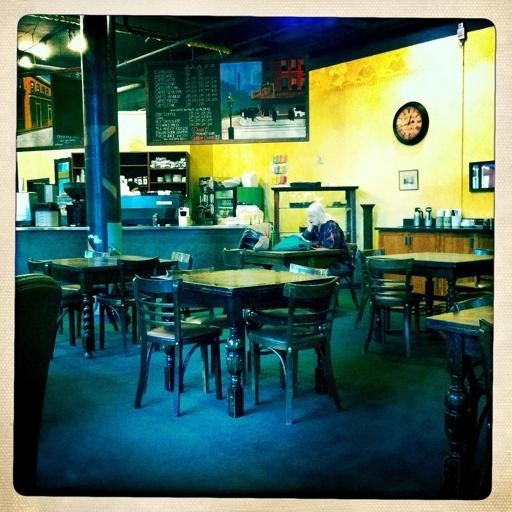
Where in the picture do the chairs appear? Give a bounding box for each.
[479,319,493,437]
[223,248,244,270]
[456,248,494,296]
[248,275,343,427]
[354,248,414,325]
[97,256,160,350]
[164,267,247,377]
[27,256,80,346]
[343,242,361,311]
[133,273,223,418]
[171,250,194,272]
[63,250,112,350]
[251,262,330,383]
[363,254,424,359]
[448,295,493,416]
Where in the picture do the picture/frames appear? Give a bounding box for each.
[469,160,495,193]
[399,169,419,191]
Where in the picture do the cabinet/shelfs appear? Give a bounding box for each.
[374,225,495,298]
[271,186,358,247]
[71,152,190,201]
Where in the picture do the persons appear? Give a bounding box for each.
[302,201,355,279]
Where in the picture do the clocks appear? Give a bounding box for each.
[394,102,429,145]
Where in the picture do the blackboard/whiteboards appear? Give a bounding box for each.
[145,52,221,146]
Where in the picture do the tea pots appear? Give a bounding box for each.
[425,207,432,227]
[414,207,423,226]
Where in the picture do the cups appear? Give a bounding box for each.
[435,217,444,229]
[152,213,159,227]
[451,216,461,228]
[443,217,452,228]
